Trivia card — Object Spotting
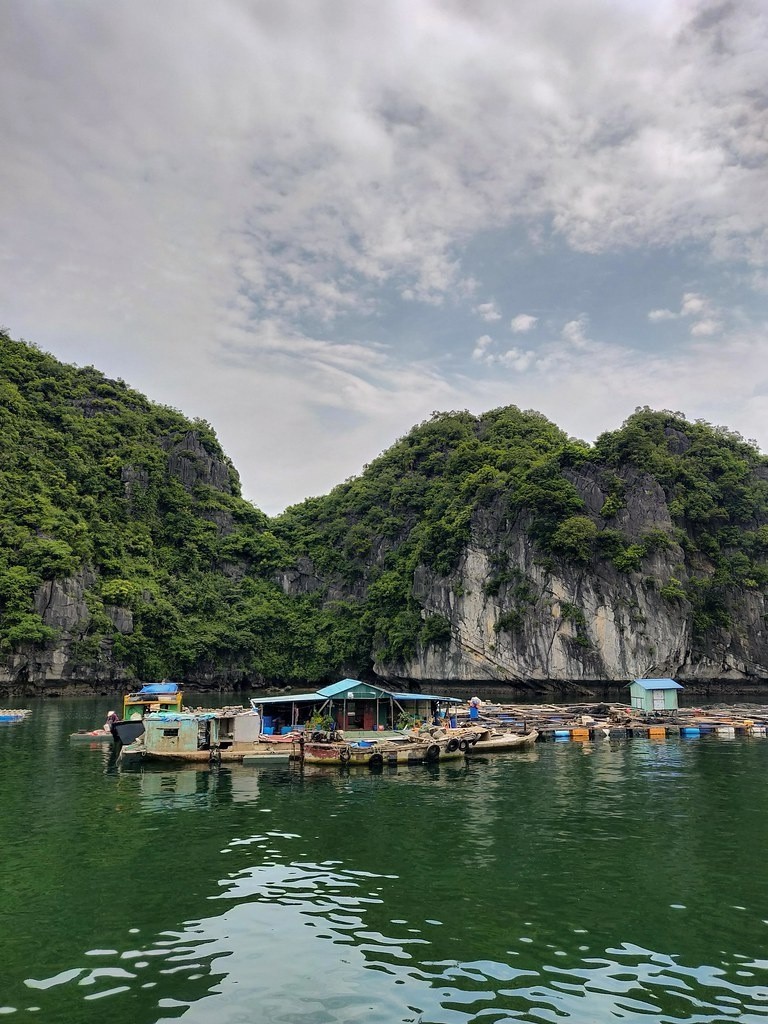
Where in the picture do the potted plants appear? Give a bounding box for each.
[304,709,334,731]
[396,711,425,729]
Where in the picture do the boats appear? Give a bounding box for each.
[0,708,33,723]
[303,727,478,766]
[470,730,540,750]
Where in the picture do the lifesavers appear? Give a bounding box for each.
[340,750,351,762]
[459,740,469,751]
[428,745,440,757]
[208,757,221,764]
[447,739,459,752]
[312,732,322,740]
